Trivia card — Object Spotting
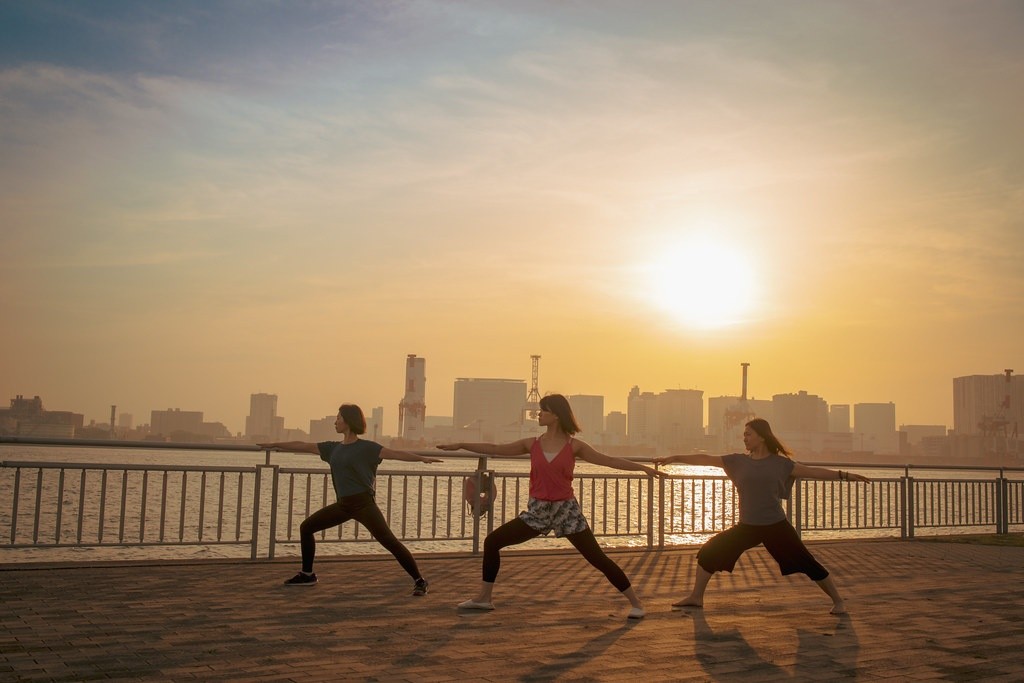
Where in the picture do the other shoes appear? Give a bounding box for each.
[627,605,644,619]
[458,599,496,610]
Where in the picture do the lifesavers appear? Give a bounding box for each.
[465,474,497,506]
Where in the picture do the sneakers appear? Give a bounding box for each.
[413,578,428,596]
[283,571,318,585]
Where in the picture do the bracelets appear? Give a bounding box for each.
[839,470,848,480]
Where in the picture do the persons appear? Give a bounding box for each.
[255,404,444,596]
[652,419,870,613]
[435,394,669,618]
